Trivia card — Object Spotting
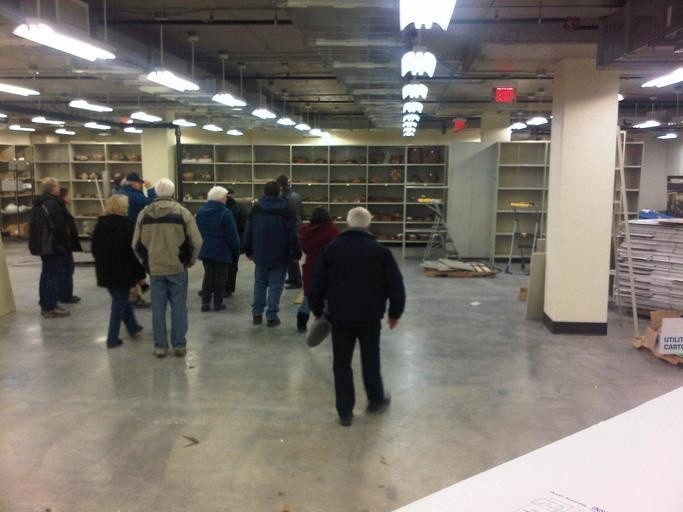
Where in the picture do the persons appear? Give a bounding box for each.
[290,207,340,329]
[275,175,303,291]
[116,171,158,305]
[305,205,407,427]
[242,179,302,329]
[128,179,203,358]
[27,177,73,318]
[194,186,243,313]
[55,188,83,303]
[198,187,245,298]
[90,194,144,349]
[111,173,124,197]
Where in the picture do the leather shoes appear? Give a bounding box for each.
[105,282,153,349]
[284,277,303,289]
[36,294,81,320]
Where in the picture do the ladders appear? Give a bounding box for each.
[418,198,459,263]
[612,126,640,340]
[505,202,541,275]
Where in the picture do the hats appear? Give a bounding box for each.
[126,172,144,182]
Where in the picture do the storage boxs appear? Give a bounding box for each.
[640,309,683,365]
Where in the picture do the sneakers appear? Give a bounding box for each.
[334,395,391,426]
[197,285,236,312]
[153,342,187,359]
[251,314,281,327]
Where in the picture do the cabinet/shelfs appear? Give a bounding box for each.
[494,142,644,266]
[0,140,142,244]
[176,141,447,246]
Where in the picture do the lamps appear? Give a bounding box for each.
[398,0,457,139]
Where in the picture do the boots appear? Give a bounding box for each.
[297,310,309,334]
